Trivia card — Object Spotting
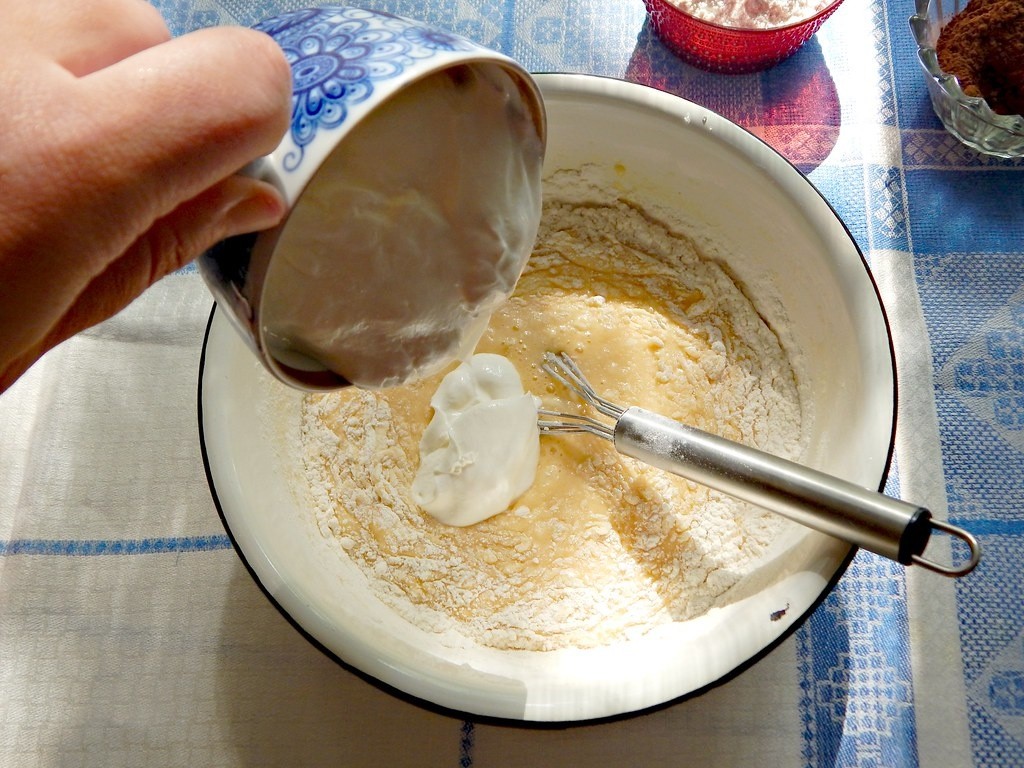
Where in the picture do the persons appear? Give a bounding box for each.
[0,0,287,397]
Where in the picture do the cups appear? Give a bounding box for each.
[147,1,546,394]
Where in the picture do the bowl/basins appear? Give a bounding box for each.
[642,1,844,71]
[195,71,898,733]
[908,1,1024,159]
